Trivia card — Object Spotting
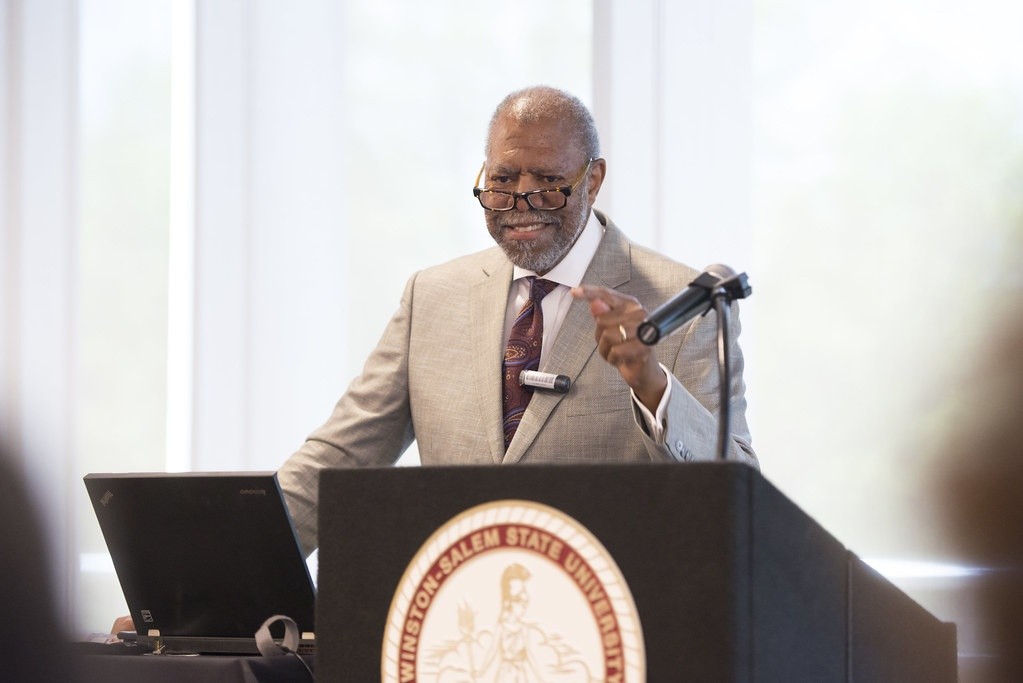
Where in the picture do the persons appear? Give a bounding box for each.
[110,86,765,638]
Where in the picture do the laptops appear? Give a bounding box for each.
[83,470,317,655]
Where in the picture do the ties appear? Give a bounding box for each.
[502,274,560,454]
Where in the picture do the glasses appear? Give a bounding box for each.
[472,160,593,213]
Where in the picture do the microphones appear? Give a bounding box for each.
[637,263,737,346]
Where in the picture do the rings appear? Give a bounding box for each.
[619,325,627,341]
